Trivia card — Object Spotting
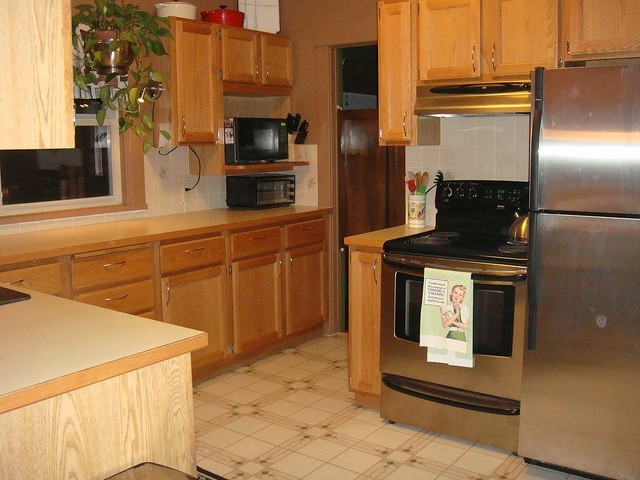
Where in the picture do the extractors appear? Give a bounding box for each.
[413,74,533,120]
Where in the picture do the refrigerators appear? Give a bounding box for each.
[516,63,639,477]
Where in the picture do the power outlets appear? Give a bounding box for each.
[182,183,198,202]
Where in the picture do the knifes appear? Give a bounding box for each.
[287,110,311,144]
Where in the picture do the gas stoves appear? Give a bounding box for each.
[378,179,528,267]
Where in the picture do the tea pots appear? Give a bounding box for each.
[505,210,529,246]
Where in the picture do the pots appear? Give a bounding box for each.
[154,0,199,20]
[200,4,246,28]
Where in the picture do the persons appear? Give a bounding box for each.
[440,285,470,342]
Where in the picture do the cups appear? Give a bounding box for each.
[406,194,426,229]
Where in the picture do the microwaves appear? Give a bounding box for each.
[224,116,289,165]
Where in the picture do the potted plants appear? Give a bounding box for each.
[71,0,171,155]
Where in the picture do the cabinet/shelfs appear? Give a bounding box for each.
[0,255,65,298]
[563,1,640,59]
[71,242,157,320]
[171,17,222,147]
[377,1,411,144]
[189,93,309,177]
[221,24,293,95]
[161,232,229,371]
[418,1,562,88]
[343,224,434,411]
[282,217,330,337]
[230,225,284,356]
[337,107,405,333]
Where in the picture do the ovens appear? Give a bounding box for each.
[379,253,519,457]
[1,97,111,208]
[225,174,296,211]
